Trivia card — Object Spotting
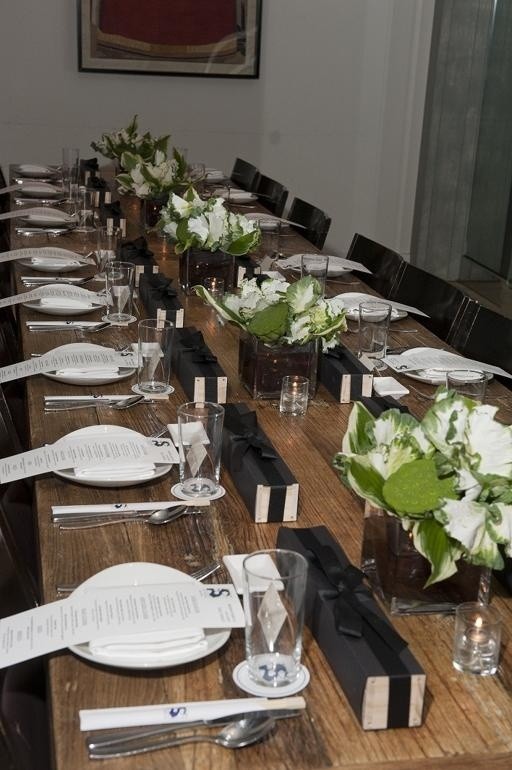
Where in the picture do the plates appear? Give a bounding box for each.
[371,376,410,399]
[40,342,136,386]
[52,424,175,487]
[62,560,233,672]
[13,164,105,317]
[287,252,353,279]
[333,291,409,323]
[399,348,493,385]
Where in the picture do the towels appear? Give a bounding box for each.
[17,167,122,381]
[413,364,482,384]
[69,464,158,486]
[87,629,211,666]
[345,304,400,319]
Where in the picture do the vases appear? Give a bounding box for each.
[177,246,239,298]
[141,193,169,229]
[358,497,495,615]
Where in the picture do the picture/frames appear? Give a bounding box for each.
[75,1,264,81]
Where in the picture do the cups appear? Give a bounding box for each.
[358,299,392,372]
[186,162,328,299]
[176,401,224,498]
[203,277,226,308]
[445,369,488,404]
[137,318,175,394]
[244,550,309,684]
[453,602,502,676]
[96,225,123,279]
[62,148,81,190]
[104,262,136,323]
[279,375,311,420]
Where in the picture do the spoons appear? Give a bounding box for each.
[89,715,276,760]
[30,320,111,332]
[59,505,189,531]
[44,395,144,413]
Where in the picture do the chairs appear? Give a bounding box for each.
[345,232,404,301]
[255,171,289,218]
[285,196,334,250]
[229,157,260,194]
[460,304,511,393]
[0,171,58,770]
[392,262,470,343]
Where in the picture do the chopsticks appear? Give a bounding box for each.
[26,321,128,326]
[52,497,211,513]
[43,393,171,401]
[81,696,307,733]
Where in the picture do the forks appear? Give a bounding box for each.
[348,326,418,334]
[57,564,219,592]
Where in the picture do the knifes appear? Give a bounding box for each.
[86,707,301,750]
[45,400,159,404]
[52,508,205,522]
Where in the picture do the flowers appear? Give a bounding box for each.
[329,384,512,592]
[111,147,192,201]
[89,109,172,173]
[191,271,351,355]
[153,185,265,258]
[237,330,320,401]
